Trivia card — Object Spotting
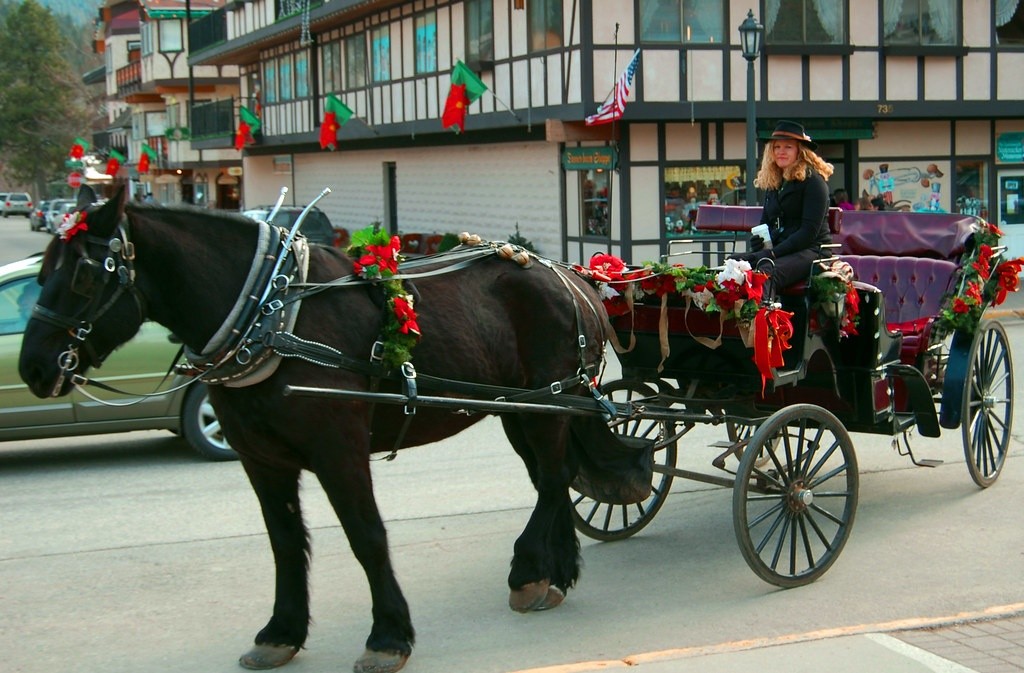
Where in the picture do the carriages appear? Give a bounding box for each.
[19,183,1014,673]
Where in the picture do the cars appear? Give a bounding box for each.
[30,200,53,231]
[52,203,77,232]
[0,192,34,218]
[43,199,76,233]
[682,186,762,222]
[665,195,691,231]
[584,198,608,219]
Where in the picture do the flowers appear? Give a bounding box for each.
[589,254,769,328]
[55,210,88,243]
[931,223,1024,334]
[344,225,421,369]
[806,275,861,339]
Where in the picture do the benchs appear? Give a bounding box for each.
[832,254,957,347]
[666,203,843,295]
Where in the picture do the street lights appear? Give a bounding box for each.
[738,9,764,251]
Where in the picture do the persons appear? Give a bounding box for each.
[143,192,156,204]
[726,120,835,303]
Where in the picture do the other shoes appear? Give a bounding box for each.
[778,351,802,370]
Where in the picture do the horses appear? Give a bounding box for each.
[14,181,611,673]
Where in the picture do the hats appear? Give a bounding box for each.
[756,120,820,151]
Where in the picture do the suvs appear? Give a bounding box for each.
[0,251,240,461]
[243,205,335,247]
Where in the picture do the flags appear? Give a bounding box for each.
[235,106,259,151]
[138,143,155,174]
[320,96,352,149]
[71,140,88,157]
[442,61,487,134]
[586,48,640,125]
[105,150,126,177]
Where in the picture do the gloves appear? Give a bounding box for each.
[727,251,772,263]
[749,227,784,251]
